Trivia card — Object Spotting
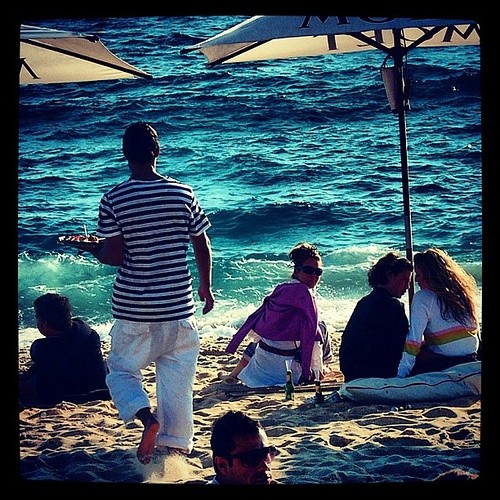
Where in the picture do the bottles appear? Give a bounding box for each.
[285,370,296,401]
[314,380,324,404]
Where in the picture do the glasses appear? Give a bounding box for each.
[294,265,323,276]
[232,446,276,469]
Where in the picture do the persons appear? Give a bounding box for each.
[396,249,480,378]
[217,243,330,389]
[18,294,112,409]
[89,122,214,465]
[339,253,418,382]
[210,411,276,485]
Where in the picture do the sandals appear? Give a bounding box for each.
[223,375,240,385]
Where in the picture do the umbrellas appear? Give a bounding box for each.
[19,23,152,85]
[179,16,481,328]
